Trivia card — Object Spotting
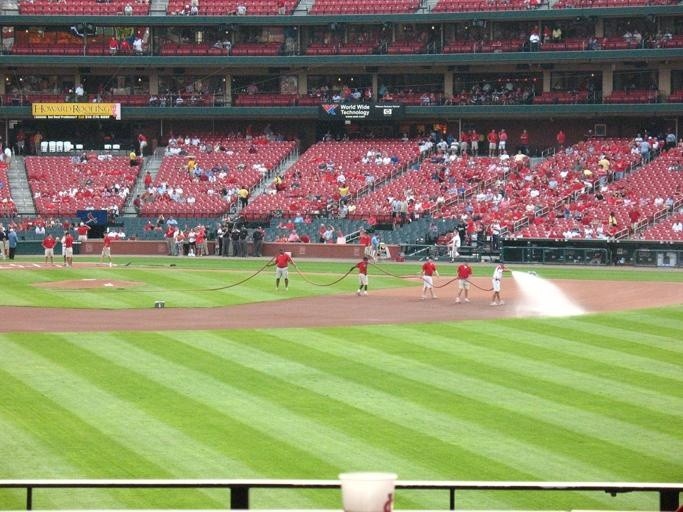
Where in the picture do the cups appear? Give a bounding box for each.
[337,471,398,512]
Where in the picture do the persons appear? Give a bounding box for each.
[417,257,439,301]
[266,248,298,291]
[355,257,369,297]
[454,261,472,303]
[490,262,506,306]
[0,0,683,270]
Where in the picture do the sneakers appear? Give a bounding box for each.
[357,289,368,296]
[455,297,471,303]
[421,295,438,299]
[489,301,503,306]
